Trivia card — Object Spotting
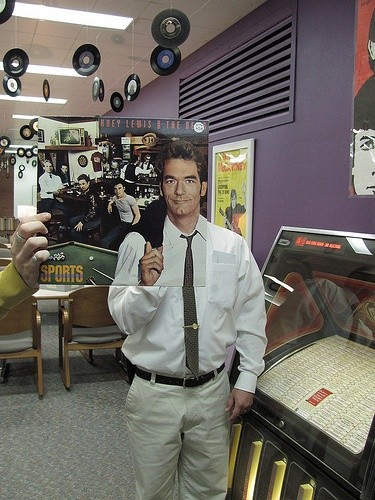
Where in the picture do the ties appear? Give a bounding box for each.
[179,230,200,381]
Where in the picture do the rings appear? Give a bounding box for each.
[31,256,41,264]
[15,232,26,243]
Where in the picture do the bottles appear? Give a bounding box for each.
[134,186,151,198]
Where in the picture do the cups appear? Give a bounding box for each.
[0,217,6,231]
[5,216,15,230]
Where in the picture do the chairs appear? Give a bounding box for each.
[58,285,137,389]
[0,295,44,395]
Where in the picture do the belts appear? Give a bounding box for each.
[135,362,226,389]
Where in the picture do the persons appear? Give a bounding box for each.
[58,164,72,184]
[58,173,98,244]
[39,160,64,216]
[138,155,153,176]
[112,139,207,285]
[99,177,141,248]
[119,155,153,196]
[107,220,268,500]
[272,264,375,341]
[0,212,51,320]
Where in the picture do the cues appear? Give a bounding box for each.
[89,278,98,285]
[92,267,114,281]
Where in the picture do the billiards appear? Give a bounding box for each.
[48,252,66,261]
[89,255,94,261]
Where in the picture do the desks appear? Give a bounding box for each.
[33,282,105,366]
